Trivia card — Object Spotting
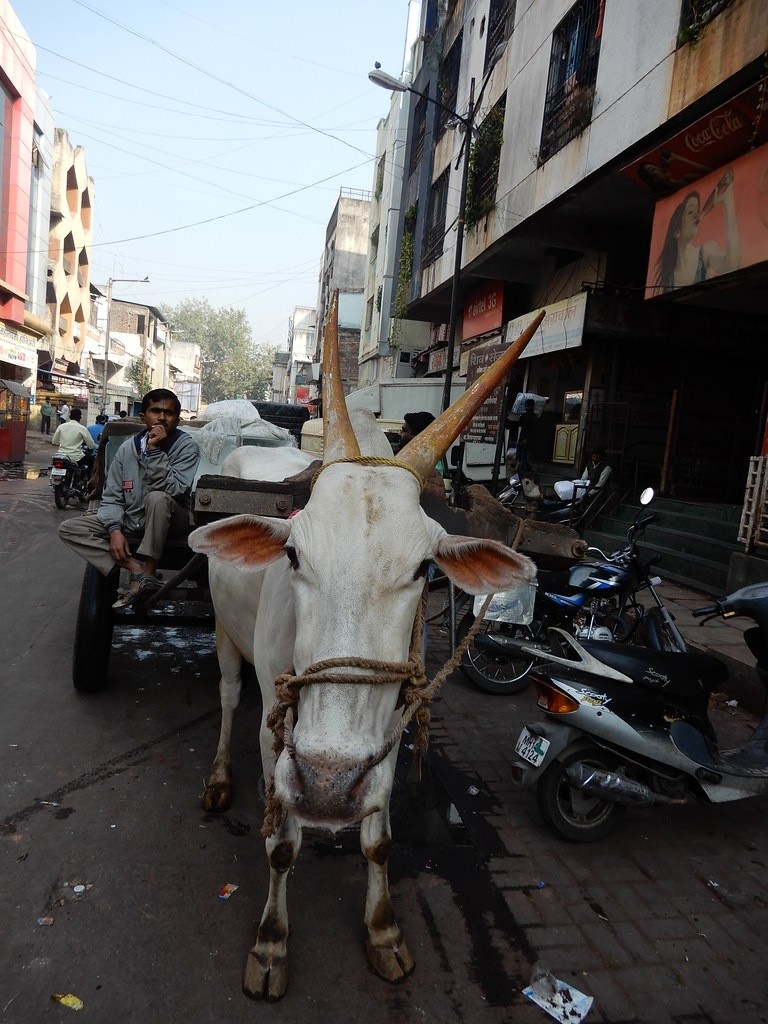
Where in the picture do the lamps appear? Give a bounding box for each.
[443,117,469,132]
[577,280,597,293]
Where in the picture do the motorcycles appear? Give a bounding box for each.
[49,444,95,510]
[455,487,687,696]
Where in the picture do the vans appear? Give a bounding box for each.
[251,401,309,450]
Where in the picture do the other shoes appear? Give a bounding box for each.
[46,432,52,434]
[41,432,45,435]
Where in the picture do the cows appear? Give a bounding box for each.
[188,289,548,1003]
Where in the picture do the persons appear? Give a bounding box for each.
[87,411,128,457]
[52,408,99,487]
[516,399,537,470]
[580,446,612,499]
[650,174,740,297]
[397,412,446,504]
[57,389,200,607]
[40,396,71,435]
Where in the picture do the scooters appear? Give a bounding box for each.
[508,581,768,844]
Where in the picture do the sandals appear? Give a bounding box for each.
[137,572,163,597]
[112,571,146,610]
